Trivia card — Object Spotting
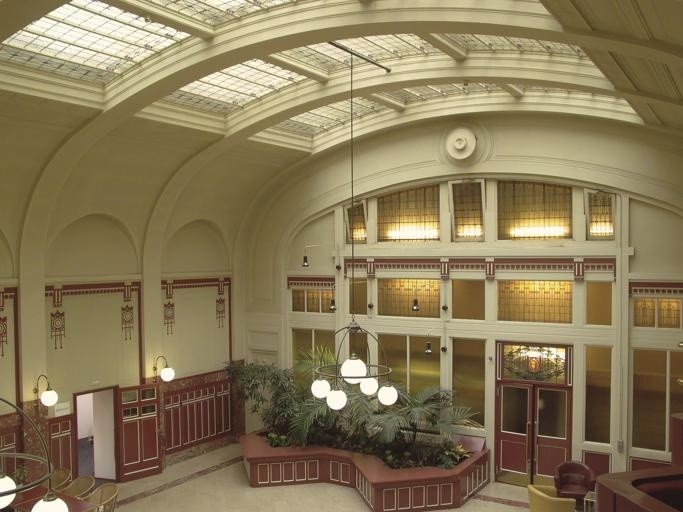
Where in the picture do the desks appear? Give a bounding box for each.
[10,488,98,512]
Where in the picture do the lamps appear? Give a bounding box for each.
[309,48,400,413]
[152,354,176,386]
[31,372,60,415]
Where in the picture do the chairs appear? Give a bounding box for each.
[526,459,597,512]
[38,465,122,512]
